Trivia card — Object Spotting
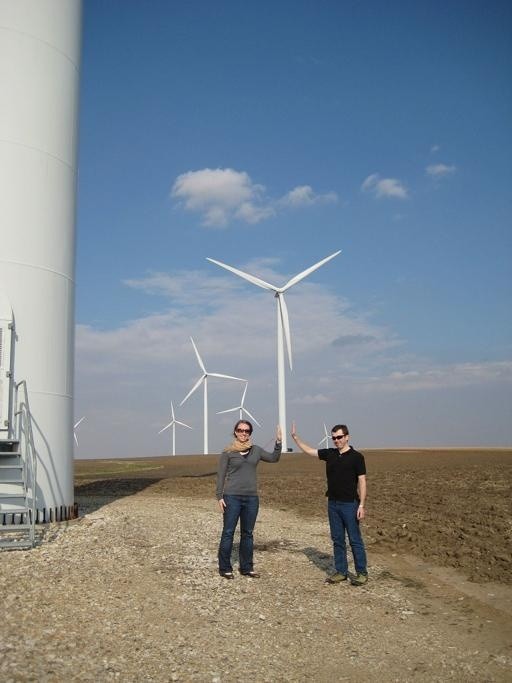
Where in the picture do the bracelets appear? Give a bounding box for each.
[358,502,365,509]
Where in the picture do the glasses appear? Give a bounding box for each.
[331,435,344,440]
[235,428,251,434]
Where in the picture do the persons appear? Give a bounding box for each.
[290,417,370,587]
[214,418,283,580]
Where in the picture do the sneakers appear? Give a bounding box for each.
[225,572,234,579]
[247,571,260,578]
[327,573,347,583]
[351,574,368,586]
